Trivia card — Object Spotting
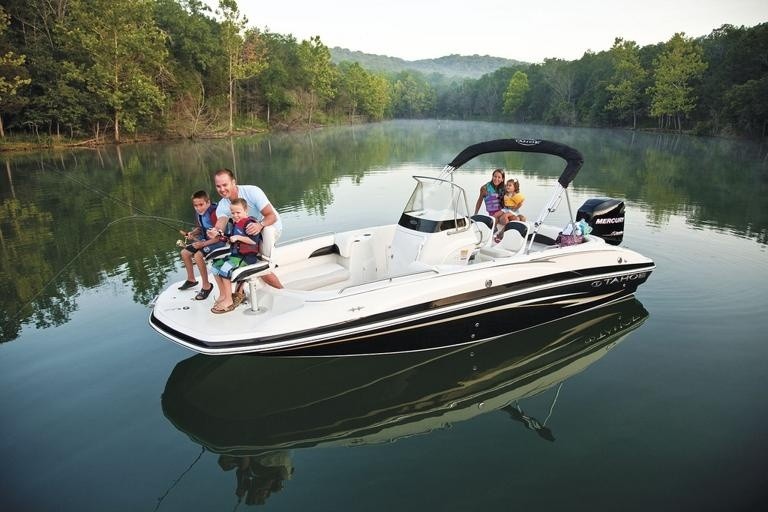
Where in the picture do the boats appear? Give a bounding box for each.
[146,135,656,359]
[159,292,649,462]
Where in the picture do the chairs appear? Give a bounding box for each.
[468,214,530,262]
[207,221,277,312]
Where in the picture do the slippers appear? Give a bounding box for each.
[177,279,198,290]
[195,283,214,300]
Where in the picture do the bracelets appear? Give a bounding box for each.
[258,220,267,228]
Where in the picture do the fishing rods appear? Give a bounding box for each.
[77,179,201,247]
[106,214,275,263]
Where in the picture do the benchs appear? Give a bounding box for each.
[277,253,351,293]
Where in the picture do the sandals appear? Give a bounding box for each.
[210,291,246,314]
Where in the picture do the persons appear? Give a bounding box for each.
[205,167,284,290]
[216,450,294,507]
[209,197,264,315]
[472,168,526,243]
[176,189,231,301]
[491,178,524,235]
